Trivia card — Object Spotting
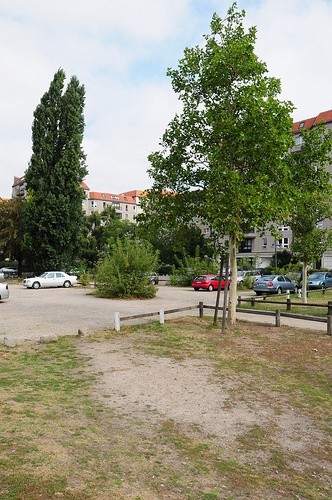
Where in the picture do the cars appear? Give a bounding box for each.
[22,271,78,289]
[192,274,231,291]
[253,275,298,295]
[307,272,332,288]
[0,283,9,300]
[144,272,159,285]
[0,267,18,273]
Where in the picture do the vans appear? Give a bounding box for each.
[227,270,262,286]
